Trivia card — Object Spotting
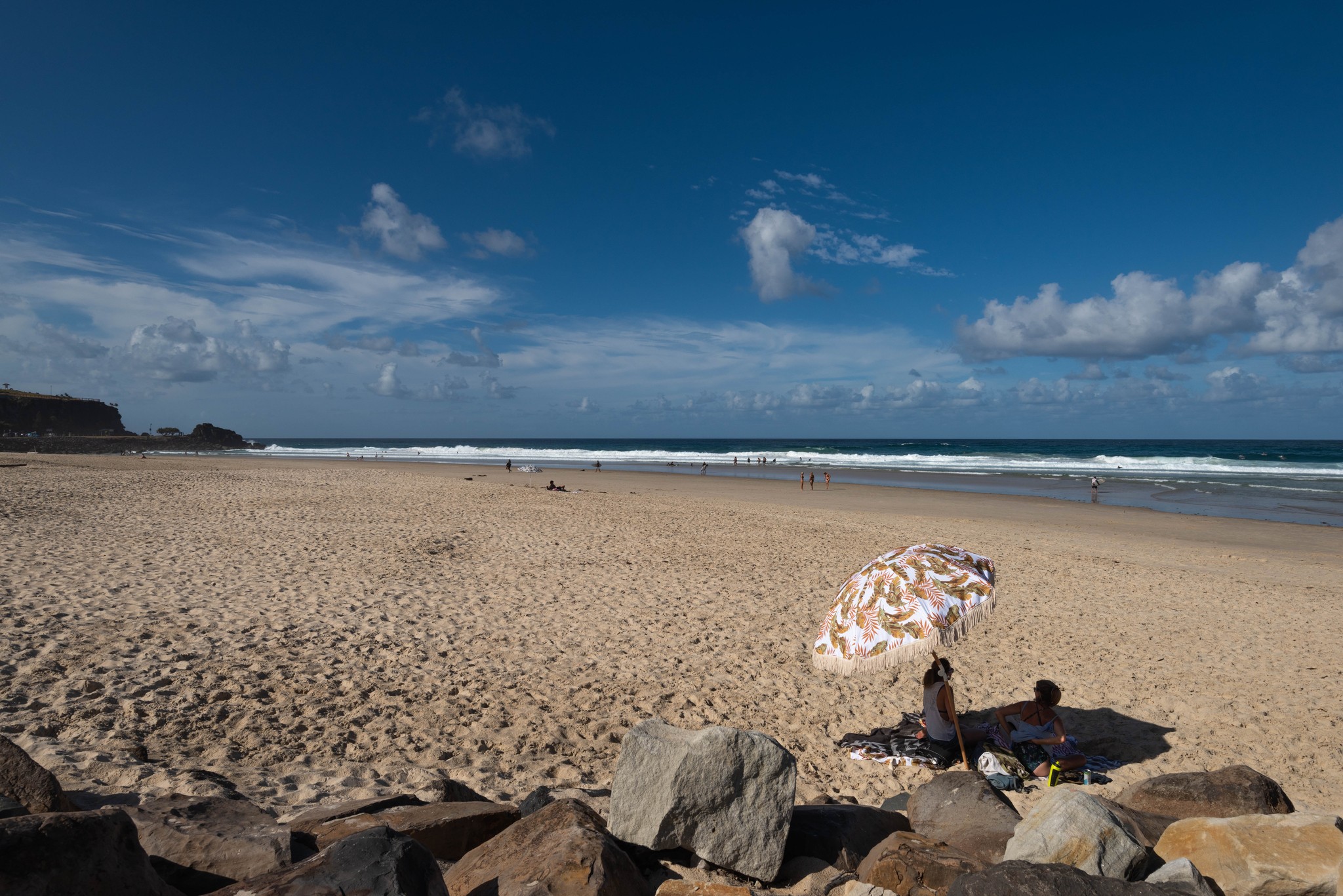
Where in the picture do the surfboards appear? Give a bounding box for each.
[418,452,420,454]
[591,464,602,467]
[700,464,708,472]
[1092,479,1107,486]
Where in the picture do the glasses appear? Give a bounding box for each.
[948,667,954,673]
[1033,687,1042,695]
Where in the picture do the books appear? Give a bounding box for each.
[999,747,1014,757]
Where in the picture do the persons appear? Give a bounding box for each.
[185,450,198,456]
[809,458,811,461]
[994,679,1087,777]
[595,460,601,472]
[702,462,706,473]
[347,451,387,460]
[121,448,147,458]
[823,472,831,489]
[800,472,804,490]
[666,462,678,466]
[916,658,986,747]
[547,481,565,491]
[809,472,814,490]
[1092,476,1099,493]
[733,456,776,465]
[799,457,802,461]
[507,459,512,473]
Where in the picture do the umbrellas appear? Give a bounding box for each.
[517,465,543,489]
[812,543,996,770]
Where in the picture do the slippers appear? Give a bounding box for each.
[959,710,970,716]
[1058,770,1084,784]
[955,710,961,716]
[1015,785,1038,794]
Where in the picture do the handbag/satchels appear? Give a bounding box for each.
[915,740,957,771]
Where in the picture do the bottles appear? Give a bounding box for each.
[1048,761,1061,787]
